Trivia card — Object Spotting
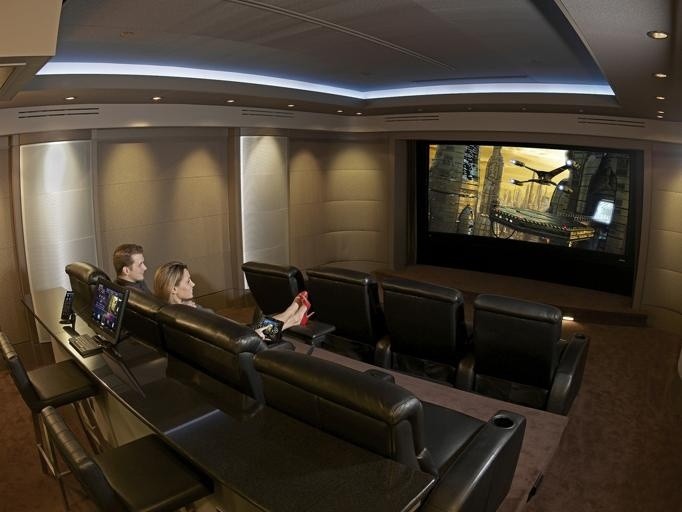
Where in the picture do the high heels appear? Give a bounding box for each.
[294,291,315,327]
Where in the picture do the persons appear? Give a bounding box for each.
[151,263,315,345]
[113,243,154,293]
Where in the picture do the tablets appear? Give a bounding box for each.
[258,317,284,340]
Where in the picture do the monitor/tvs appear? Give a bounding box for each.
[97,347,145,399]
[87,279,130,343]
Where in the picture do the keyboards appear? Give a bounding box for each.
[68,335,100,357]
